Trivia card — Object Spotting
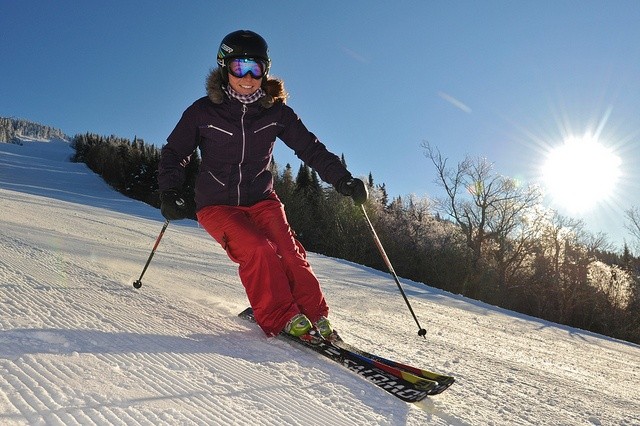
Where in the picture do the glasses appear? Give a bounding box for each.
[217,55,271,80]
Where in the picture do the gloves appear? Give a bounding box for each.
[159,189,187,220]
[338,175,369,205]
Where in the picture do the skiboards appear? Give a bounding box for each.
[239,307,454,403]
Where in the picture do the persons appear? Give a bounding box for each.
[157,30,367,338]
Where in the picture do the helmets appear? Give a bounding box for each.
[217,30,272,91]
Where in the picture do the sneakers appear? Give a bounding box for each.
[313,316,333,338]
[282,313,313,336]
